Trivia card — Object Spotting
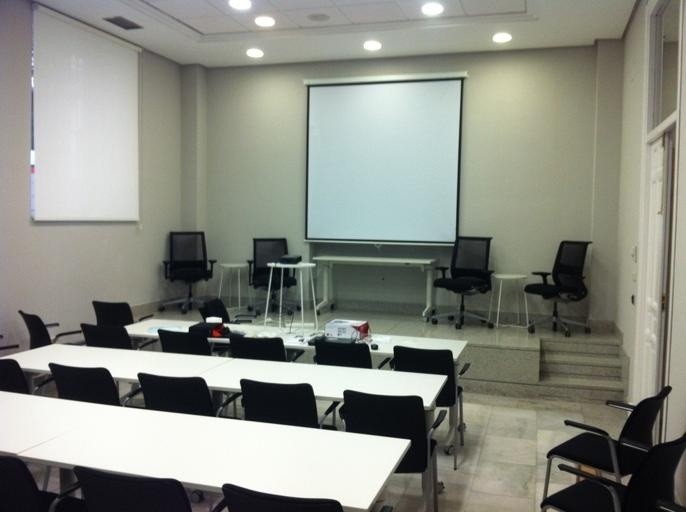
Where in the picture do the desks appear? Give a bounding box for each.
[311,255,440,323]
[0,343,459,497]
[0,389,412,512]
[125,318,469,427]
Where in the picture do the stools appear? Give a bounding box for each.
[264,262,318,334]
[487,273,530,329]
[218,263,252,312]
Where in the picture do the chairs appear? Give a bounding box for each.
[0,358,54,395]
[313,341,391,370]
[524,240,593,337]
[137,372,242,417]
[229,335,305,362]
[430,236,494,329]
[542,385,672,498]
[48,363,143,406]
[212,483,343,512]
[389,345,471,445]
[72,465,192,512]
[0,455,80,512]
[92,300,154,326]
[246,237,301,316]
[80,323,157,350]
[158,231,217,314]
[157,329,212,356]
[240,378,340,431]
[18,310,82,350]
[539,431,686,512]
[339,389,447,494]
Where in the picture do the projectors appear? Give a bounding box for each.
[325,319,368,341]
